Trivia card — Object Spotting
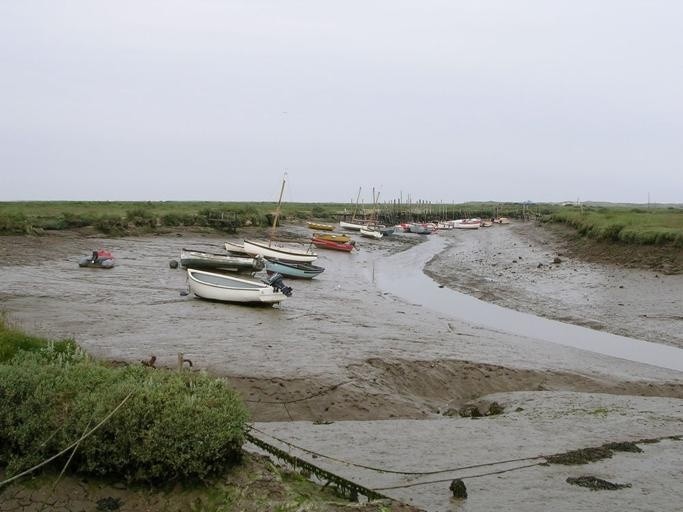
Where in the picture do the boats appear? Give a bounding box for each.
[80,250,114,267]
[306,221,337,231]
[186,268,293,304]
[312,239,360,253]
[312,231,351,243]
[181,238,326,280]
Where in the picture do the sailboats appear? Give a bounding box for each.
[392,191,510,234]
[340,186,394,240]
